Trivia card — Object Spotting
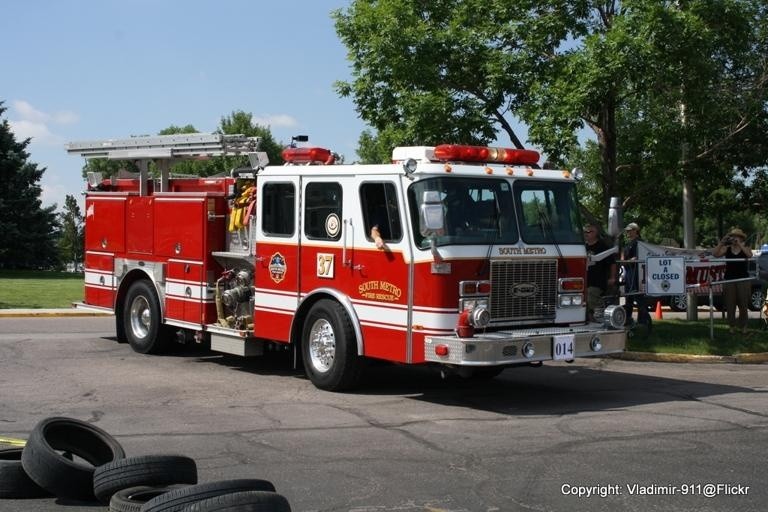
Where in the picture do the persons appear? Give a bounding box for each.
[621,222,654,324]
[713,227,754,335]
[584,224,617,320]
[369,224,391,254]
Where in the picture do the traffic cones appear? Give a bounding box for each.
[652,301,664,321]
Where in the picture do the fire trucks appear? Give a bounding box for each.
[63,129,630,392]
[615,241,767,316]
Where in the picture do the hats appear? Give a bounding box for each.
[728,229,747,240]
[624,223,640,231]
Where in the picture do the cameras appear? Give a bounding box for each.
[724,236,735,246]
